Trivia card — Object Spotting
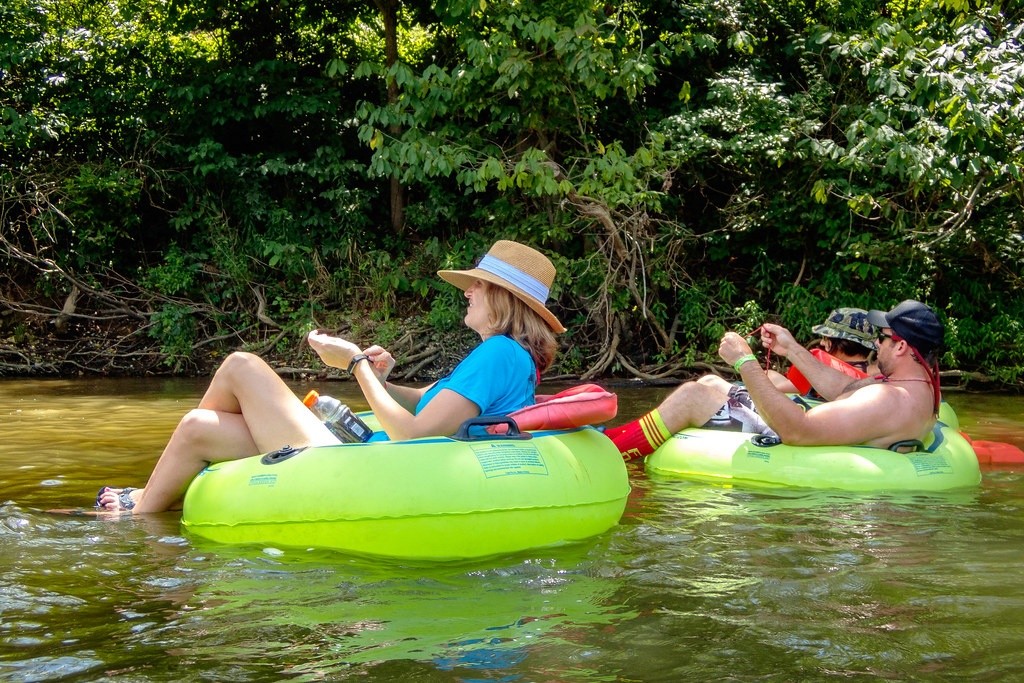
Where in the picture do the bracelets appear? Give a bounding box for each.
[347,353,368,376]
[733,354,757,373]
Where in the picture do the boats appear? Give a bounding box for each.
[731,373,963,434]
[639,414,984,492]
[178,383,634,568]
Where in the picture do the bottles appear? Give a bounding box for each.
[303,389,374,444]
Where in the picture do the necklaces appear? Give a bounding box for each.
[882,379,933,383]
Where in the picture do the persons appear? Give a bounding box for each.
[603,298,945,463]
[95,239,568,516]
[763,306,880,403]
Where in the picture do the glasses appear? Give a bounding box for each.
[877,328,891,344]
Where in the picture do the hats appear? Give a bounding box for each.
[867,299,944,353]
[812,307,880,351]
[437,240,568,334]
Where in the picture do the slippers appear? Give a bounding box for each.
[97,486,184,523]
